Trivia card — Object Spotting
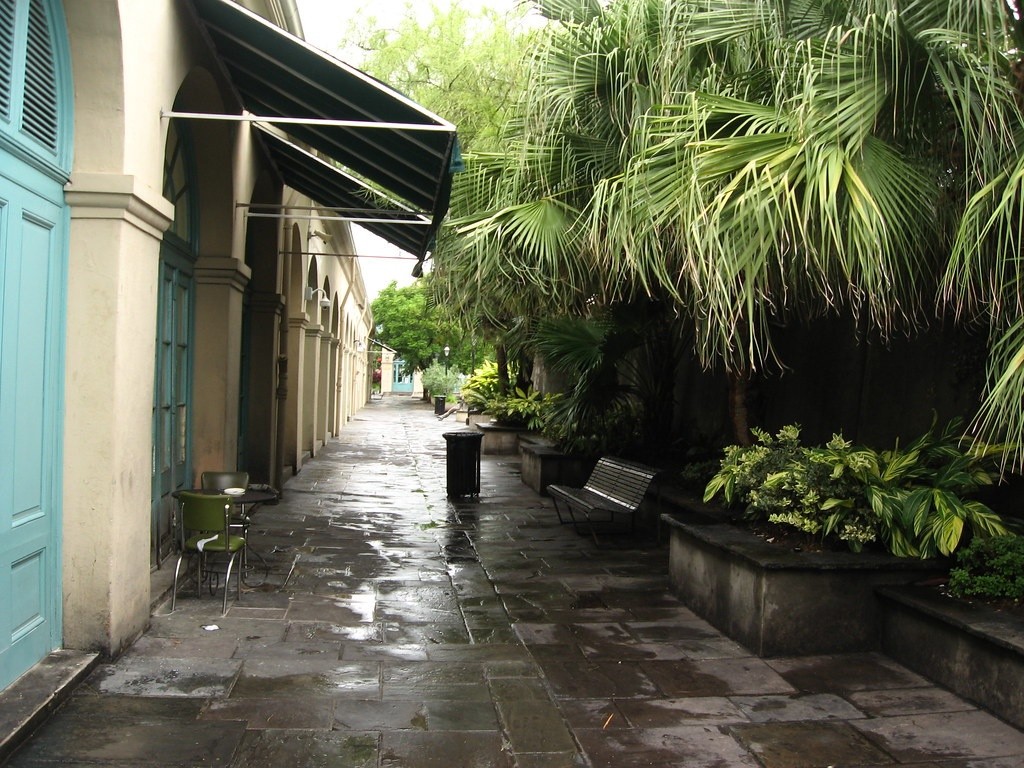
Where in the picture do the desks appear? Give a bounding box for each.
[171,487,277,594]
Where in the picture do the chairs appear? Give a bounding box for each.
[200,471,250,578]
[171,491,247,614]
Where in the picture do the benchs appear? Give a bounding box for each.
[546,456,661,549]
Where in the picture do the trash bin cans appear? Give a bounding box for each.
[441,432,486,500]
[434,395,446,415]
[422,385,428,399]
